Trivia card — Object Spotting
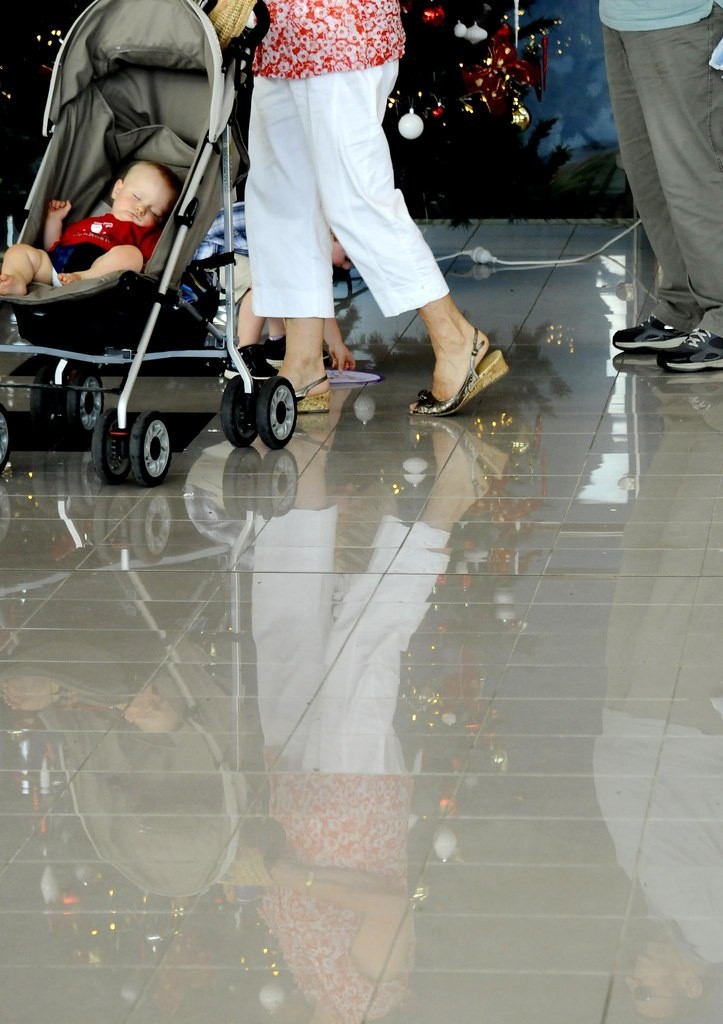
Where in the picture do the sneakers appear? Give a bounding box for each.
[264,336,329,367]
[612,315,689,354]
[657,329,723,373]
[224,344,279,380]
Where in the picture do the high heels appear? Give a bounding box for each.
[409,326,510,417]
[295,374,329,414]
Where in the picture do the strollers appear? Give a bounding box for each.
[0,0,298,487]
[0,446,298,899]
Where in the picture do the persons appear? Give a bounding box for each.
[245,0,511,418]
[597,0,723,374]
[192,201,355,386]
[0,159,182,296]
